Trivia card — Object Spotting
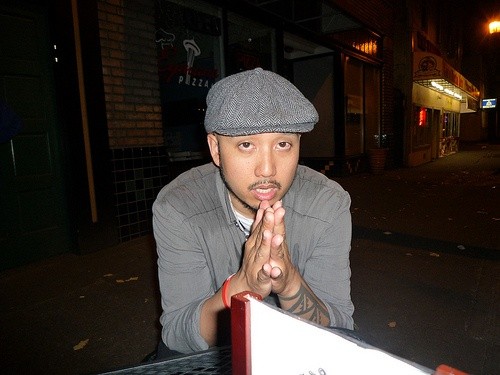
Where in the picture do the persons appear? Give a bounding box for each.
[150,67,356,364]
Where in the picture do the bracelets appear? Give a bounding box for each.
[221,272,237,312]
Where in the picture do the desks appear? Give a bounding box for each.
[97,326,384,375]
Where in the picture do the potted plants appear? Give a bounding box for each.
[367,133,391,171]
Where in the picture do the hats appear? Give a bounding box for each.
[206,68,319,134]
